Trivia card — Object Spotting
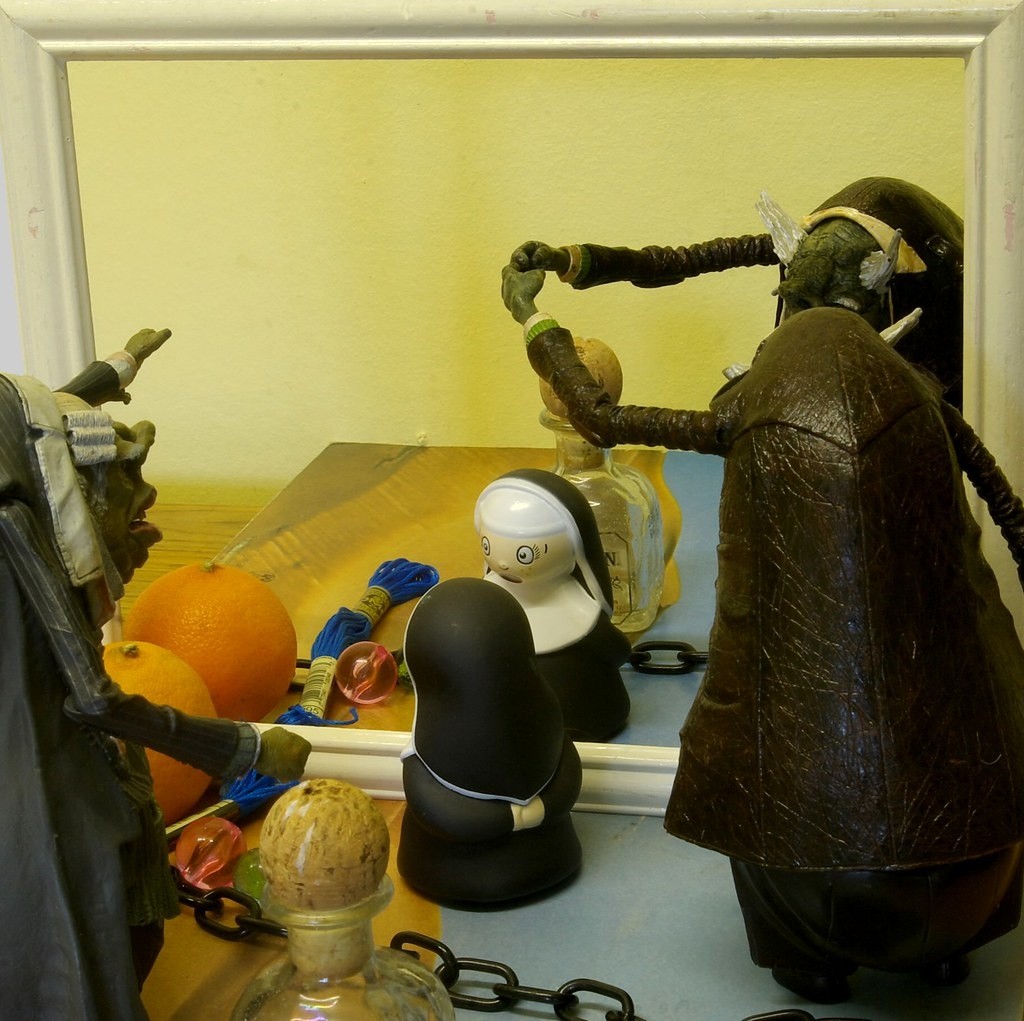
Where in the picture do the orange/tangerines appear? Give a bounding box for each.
[103,556,299,825]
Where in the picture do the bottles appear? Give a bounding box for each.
[223,875,459,1021]
[534,407,661,638]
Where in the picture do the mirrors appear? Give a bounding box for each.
[0,1,1024,822]
